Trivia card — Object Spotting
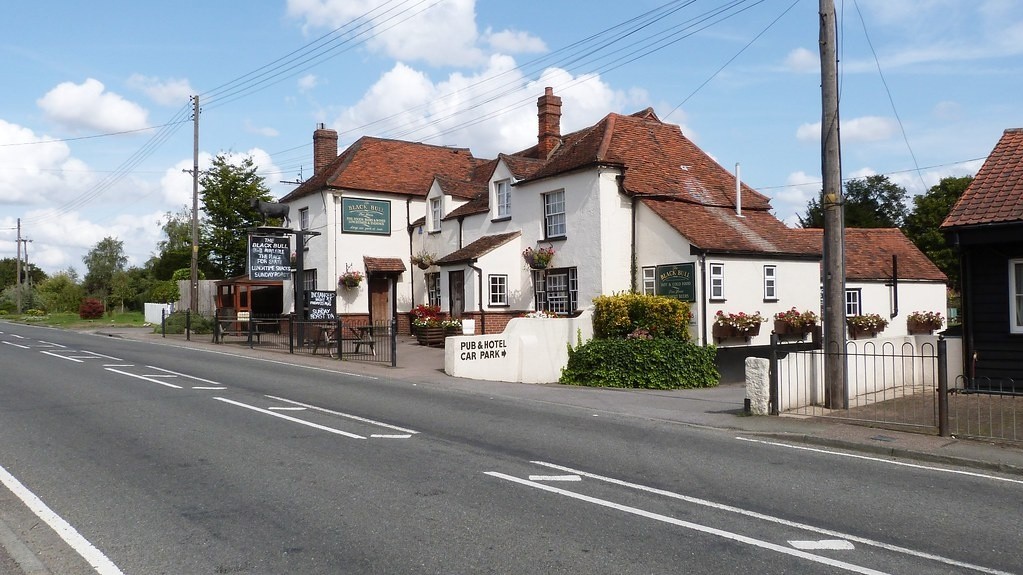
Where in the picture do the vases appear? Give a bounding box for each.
[907,323,937,335]
[849,325,883,339]
[530,259,548,269]
[415,326,463,347]
[713,324,760,337]
[774,320,813,335]
[346,282,358,287]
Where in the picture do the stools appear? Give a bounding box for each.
[357,325,376,356]
[317,340,337,358]
[353,340,377,355]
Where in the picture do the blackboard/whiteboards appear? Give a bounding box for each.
[308,290,337,320]
[248,235,292,281]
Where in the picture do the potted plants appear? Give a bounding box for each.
[413,253,436,269]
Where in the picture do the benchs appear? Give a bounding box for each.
[216,321,262,341]
[313,325,370,358]
[218,330,265,344]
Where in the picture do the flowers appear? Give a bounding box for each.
[846,314,889,330]
[774,306,819,328]
[908,311,946,327]
[716,310,767,332]
[339,272,364,290]
[409,306,462,328]
[522,247,555,266]
